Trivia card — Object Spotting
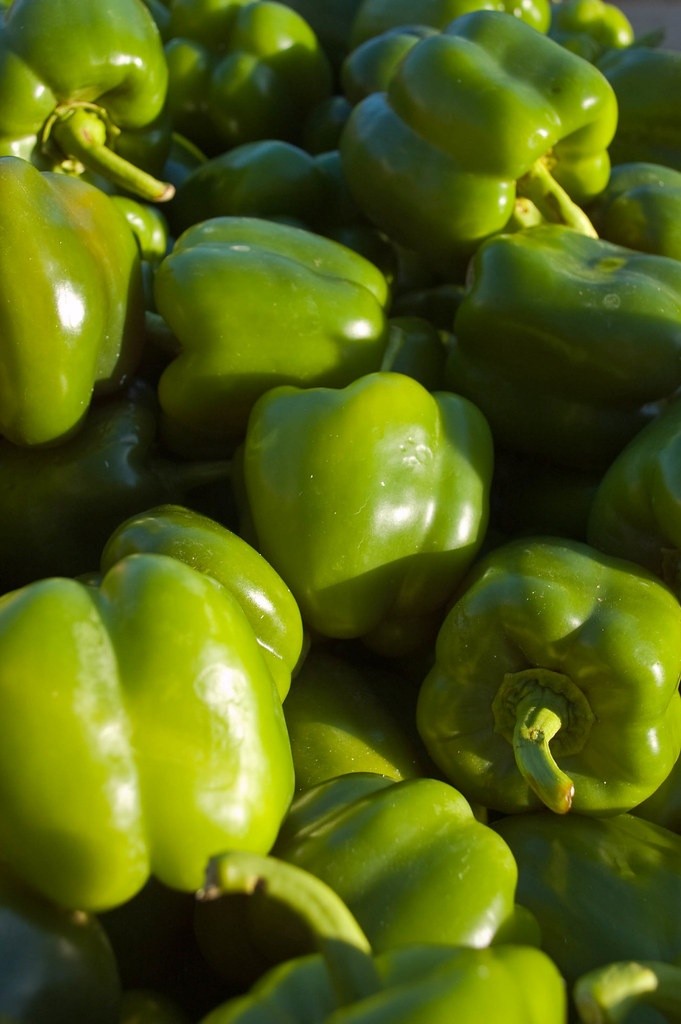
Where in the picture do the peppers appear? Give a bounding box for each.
[0,0,681,1024]
